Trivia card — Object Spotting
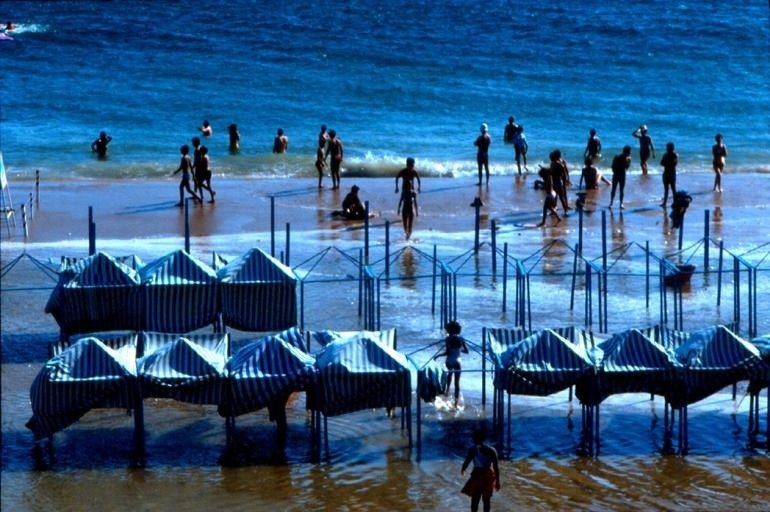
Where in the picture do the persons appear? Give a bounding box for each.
[474,123,491,186]
[578,130,611,189]
[712,134,727,193]
[92,132,111,161]
[315,124,343,190]
[229,124,240,154]
[535,149,572,226]
[433,321,469,408]
[660,142,678,207]
[273,128,288,154]
[632,126,656,175]
[609,146,631,209]
[173,138,216,205]
[3,22,16,32]
[334,185,375,221]
[505,118,529,176]
[396,158,422,232]
[199,121,212,136]
[458,426,501,512]
